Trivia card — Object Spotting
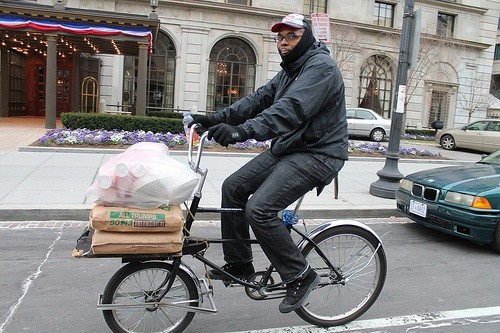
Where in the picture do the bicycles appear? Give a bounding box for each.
[95,121,388,333]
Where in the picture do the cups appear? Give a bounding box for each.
[87,157,153,204]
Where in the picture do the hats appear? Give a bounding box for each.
[271,13,308,32]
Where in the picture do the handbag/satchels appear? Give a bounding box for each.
[86,142,200,209]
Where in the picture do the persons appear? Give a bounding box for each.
[184,13,348,314]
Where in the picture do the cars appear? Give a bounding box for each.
[434,119,500,154]
[345,107,392,142]
[394,149,500,254]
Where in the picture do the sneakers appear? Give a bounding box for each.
[205,261,256,281]
[279,265,321,313]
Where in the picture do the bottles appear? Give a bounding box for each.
[182,111,199,144]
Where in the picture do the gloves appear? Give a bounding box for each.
[188,114,220,137]
[207,123,247,148]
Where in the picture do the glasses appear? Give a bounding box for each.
[274,32,303,43]
